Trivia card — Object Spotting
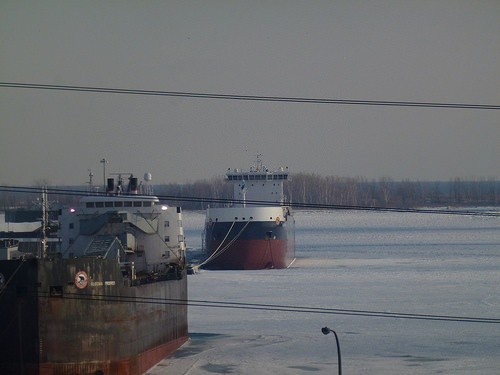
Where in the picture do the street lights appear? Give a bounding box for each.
[321,327,343,375]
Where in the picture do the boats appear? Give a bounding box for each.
[0,158,198,375]
[194,151,293,269]
